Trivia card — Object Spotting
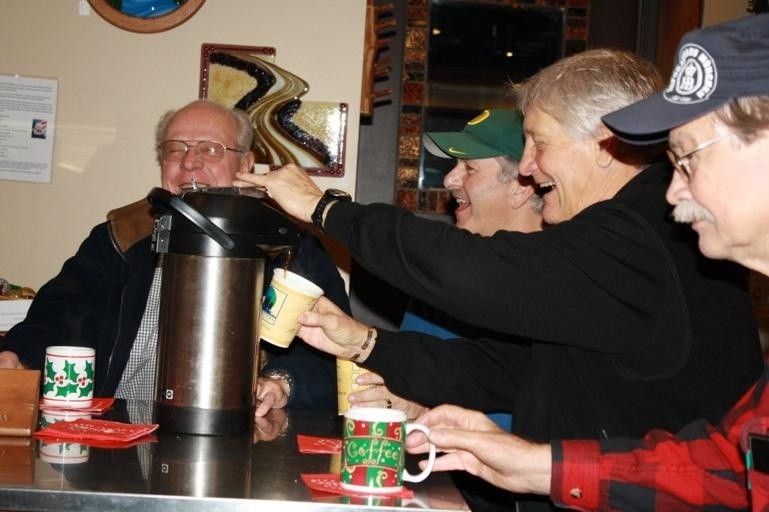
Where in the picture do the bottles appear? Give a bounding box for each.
[147,185,300,438]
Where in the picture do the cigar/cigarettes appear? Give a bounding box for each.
[190,178,197,190]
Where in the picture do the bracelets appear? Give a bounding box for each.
[350,326,373,362]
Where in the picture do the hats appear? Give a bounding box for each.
[423,107,525,161]
[601,13,766,145]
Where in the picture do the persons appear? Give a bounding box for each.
[0,100,353,418]
[233,47,764,512]
[406,13,769,512]
[349,107,544,433]
[253,409,292,444]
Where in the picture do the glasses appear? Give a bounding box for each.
[158,140,243,162]
[666,134,718,181]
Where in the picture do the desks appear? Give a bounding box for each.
[0,399,471,511]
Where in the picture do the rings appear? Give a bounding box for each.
[385,399,392,409]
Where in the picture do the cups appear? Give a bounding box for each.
[42,345,96,409]
[333,353,381,418]
[258,268,325,350]
[39,408,92,464]
[340,408,436,495]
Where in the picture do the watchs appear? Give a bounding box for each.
[311,189,351,232]
[275,416,290,440]
[261,368,291,398]
